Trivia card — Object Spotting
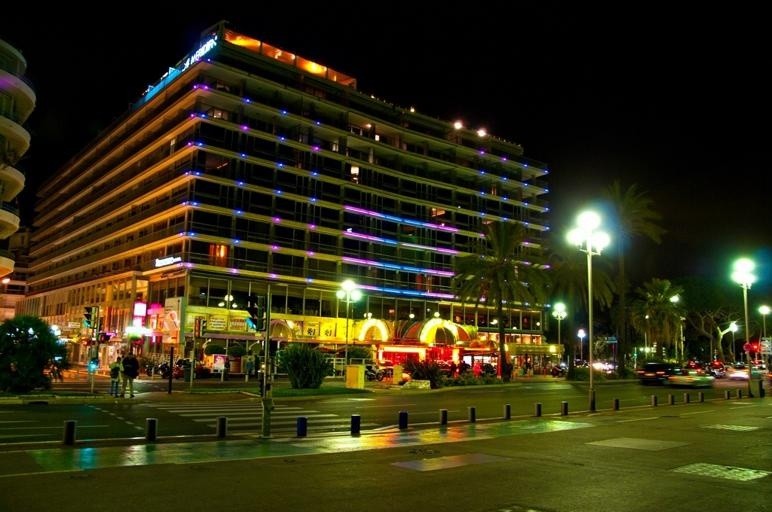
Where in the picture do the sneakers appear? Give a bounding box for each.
[110,393,134,398]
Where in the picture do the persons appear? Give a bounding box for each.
[450,360,470,374]
[109,352,139,398]
[246,355,261,378]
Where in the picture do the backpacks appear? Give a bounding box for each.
[110,363,120,379]
[123,364,134,376]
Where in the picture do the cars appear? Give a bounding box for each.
[551,358,618,379]
[421,359,501,385]
[634,357,772,391]
[173,357,207,379]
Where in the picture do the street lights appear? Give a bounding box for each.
[577,328,586,361]
[551,302,568,367]
[218,294,238,310]
[566,205,613,411]
[729,256,757,394]
[758,305,770,373]
[336,279,364,381]
[729,323,738,364]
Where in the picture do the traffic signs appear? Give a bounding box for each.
[761,337,772,341]
[761,342,772,347]
[760,348,771,351]
[760,351,772,355]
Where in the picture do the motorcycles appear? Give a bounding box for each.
[364,361,385,382]
[142,360,171,380]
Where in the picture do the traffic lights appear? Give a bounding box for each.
[83,306,96,329]
[80,339,91,346]
[98,334,110,344]
[246,294,264,331]
[194,314,207,339]
[88,358,100,374]
